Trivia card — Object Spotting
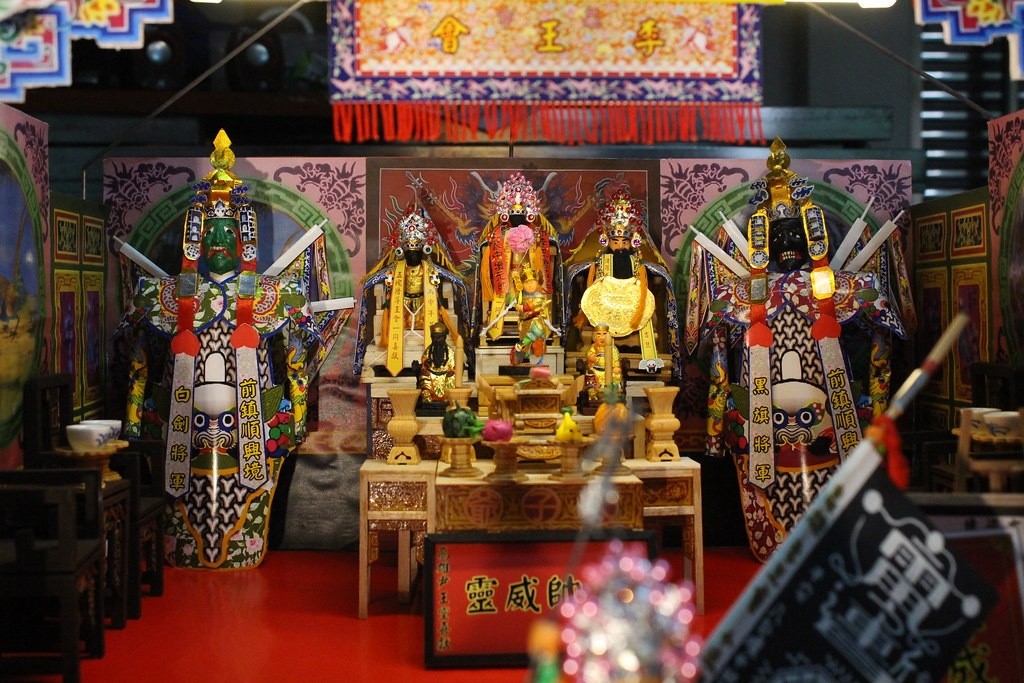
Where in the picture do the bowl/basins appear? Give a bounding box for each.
[983,410,1020,440]
[66,425,110,450]
[79,419,122,440]
[960,408,1001,431]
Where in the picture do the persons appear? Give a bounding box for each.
[681,133,921,565]
[565,188,683,385]
[506,262,553,360]
[113,129,326,570]
[470,172,568,341]
[584,323,627,400]
[412,322,456,404]
[352,206,476,377]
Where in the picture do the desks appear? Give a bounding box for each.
[98,478,129,641]
[359,458,703,617]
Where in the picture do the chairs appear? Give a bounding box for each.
[22,373,165,620]
[0,467,99,683]
